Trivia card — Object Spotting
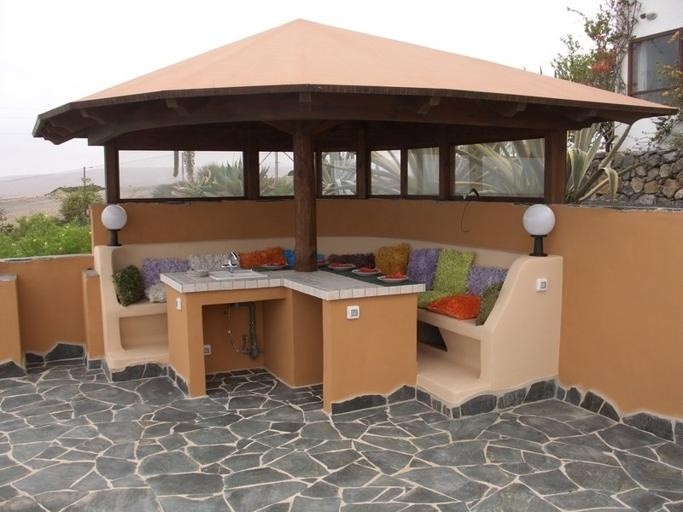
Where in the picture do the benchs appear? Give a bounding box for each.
[92,233,564,406]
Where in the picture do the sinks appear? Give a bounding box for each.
[208,268,268,281]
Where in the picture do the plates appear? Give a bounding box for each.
[318,259,330,267]
[328,263,409,284]
[259,262,290,270]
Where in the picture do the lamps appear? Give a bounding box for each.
[522,204,557,257]
[100,204,127,248]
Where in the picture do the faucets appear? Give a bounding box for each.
[226,251,239,267]
